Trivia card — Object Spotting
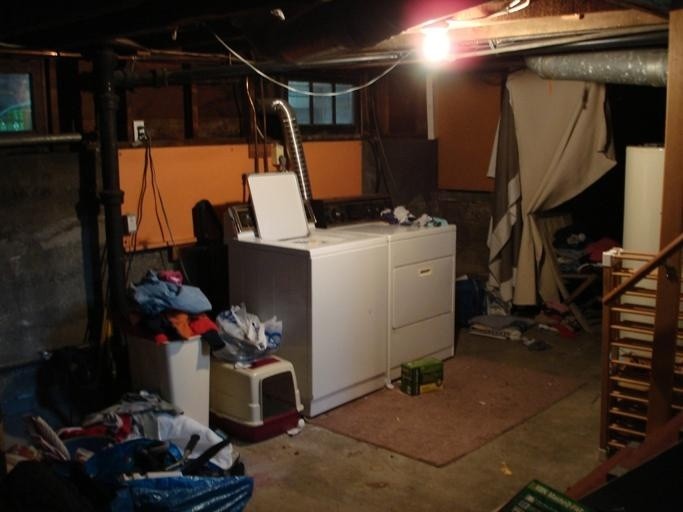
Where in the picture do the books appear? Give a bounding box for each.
[495,478,589,512]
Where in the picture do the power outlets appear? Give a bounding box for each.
[133,120,145,142]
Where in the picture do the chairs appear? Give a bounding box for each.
[527,211,604,336]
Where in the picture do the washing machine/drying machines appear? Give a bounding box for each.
[223,172,388,418]
[312,193,456,383]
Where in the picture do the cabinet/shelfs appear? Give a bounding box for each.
[388,225,456,383]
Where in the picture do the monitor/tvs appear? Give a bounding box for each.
[0,71,37,136]
